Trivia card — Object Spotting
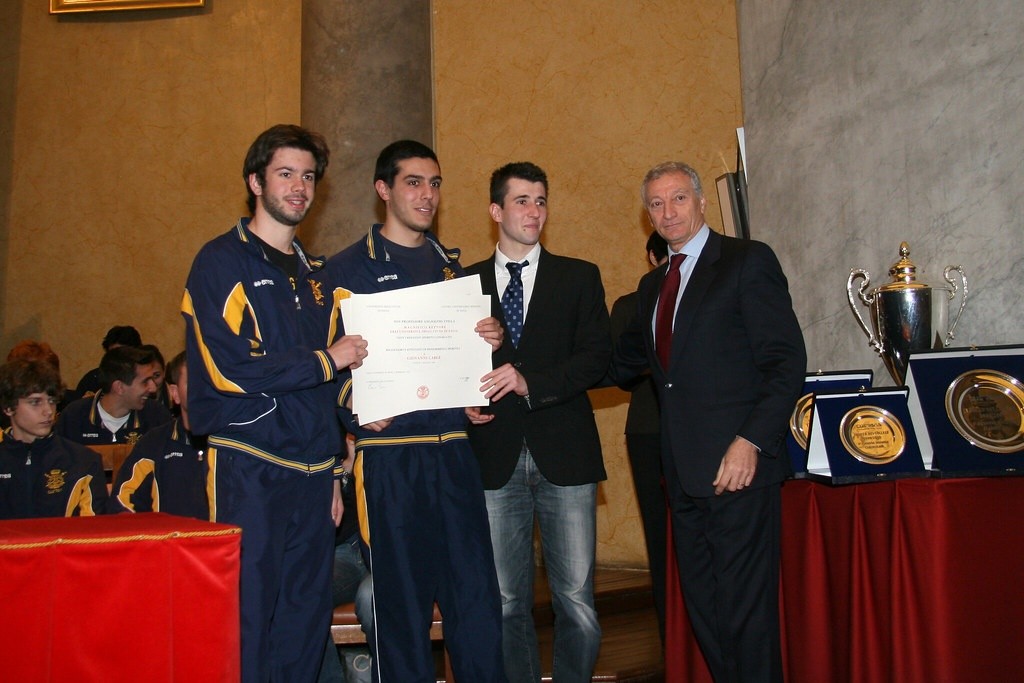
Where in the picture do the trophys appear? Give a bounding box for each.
[846,241,969,387]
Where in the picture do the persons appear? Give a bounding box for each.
[318,435,377,683]
[610,230,670,640]
[462,161,613,683]
[0,357,109,519]
[593,160,807,683]
[108,348,208,519]
[319,138,507,683]
[0,323,181,445]
[181,125,367,683]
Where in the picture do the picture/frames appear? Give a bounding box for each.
[49,0,206,15]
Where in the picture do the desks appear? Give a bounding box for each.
[0,511,243,683]
[660,466,1024,683]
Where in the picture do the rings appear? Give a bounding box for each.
[738,483,745,486]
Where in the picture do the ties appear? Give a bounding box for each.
[655,253,689,379]
[499,258,529,348]
